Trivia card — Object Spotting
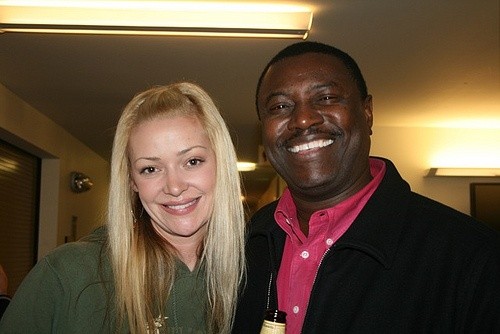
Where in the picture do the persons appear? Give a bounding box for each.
[228,37,500,334]
[0,81,250,334]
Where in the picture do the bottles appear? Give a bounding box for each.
[260,310,287,334]
